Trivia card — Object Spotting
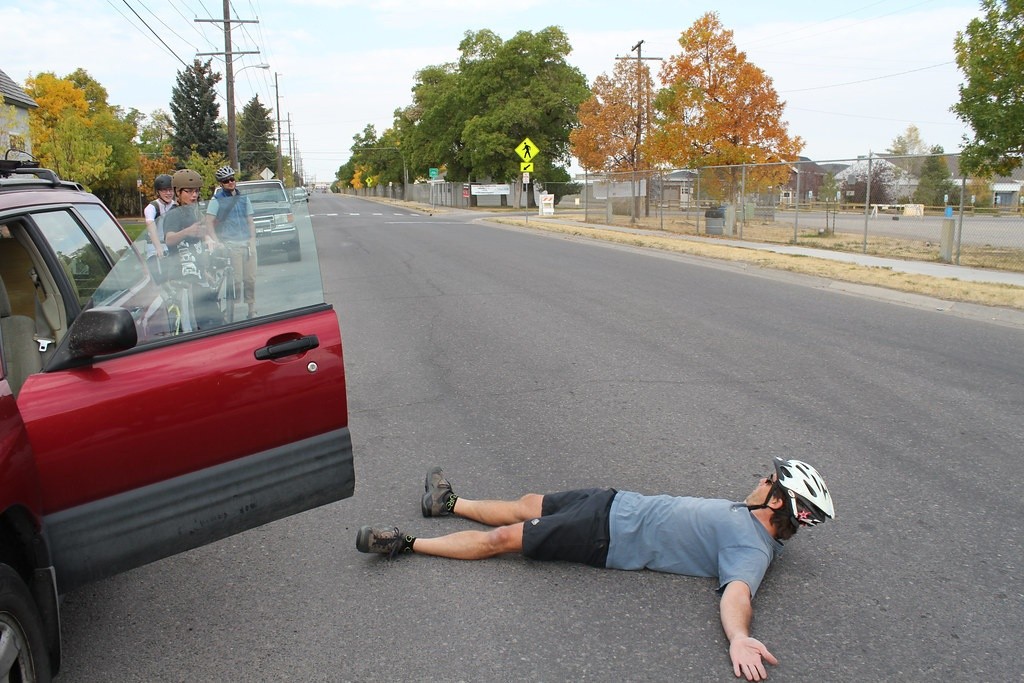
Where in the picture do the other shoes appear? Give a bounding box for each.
[246,310,258,320]
[222,303,242,317]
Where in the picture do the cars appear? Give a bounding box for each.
[291,186,312,203]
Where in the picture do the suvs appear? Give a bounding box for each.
[204,180,300,261]
[0,145,355,683]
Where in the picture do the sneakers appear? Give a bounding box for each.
[422,466,455,517]
[356,526,403,564]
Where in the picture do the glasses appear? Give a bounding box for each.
[182,188,200,194]
[220,177,236,185]
[158,187,174,193]
[765,473,778,491]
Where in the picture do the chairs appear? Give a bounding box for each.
[0,275,43,399]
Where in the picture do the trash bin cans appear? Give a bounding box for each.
[705,209,724,235]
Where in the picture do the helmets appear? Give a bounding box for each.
[154,174,173,189]
[171,169,204,191]
[774,455,834,526]
[215,166,235,181]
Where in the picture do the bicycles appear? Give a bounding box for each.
[211,238,257,326]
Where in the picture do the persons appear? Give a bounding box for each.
[144,165,257,329]
[356,457,835,681]
